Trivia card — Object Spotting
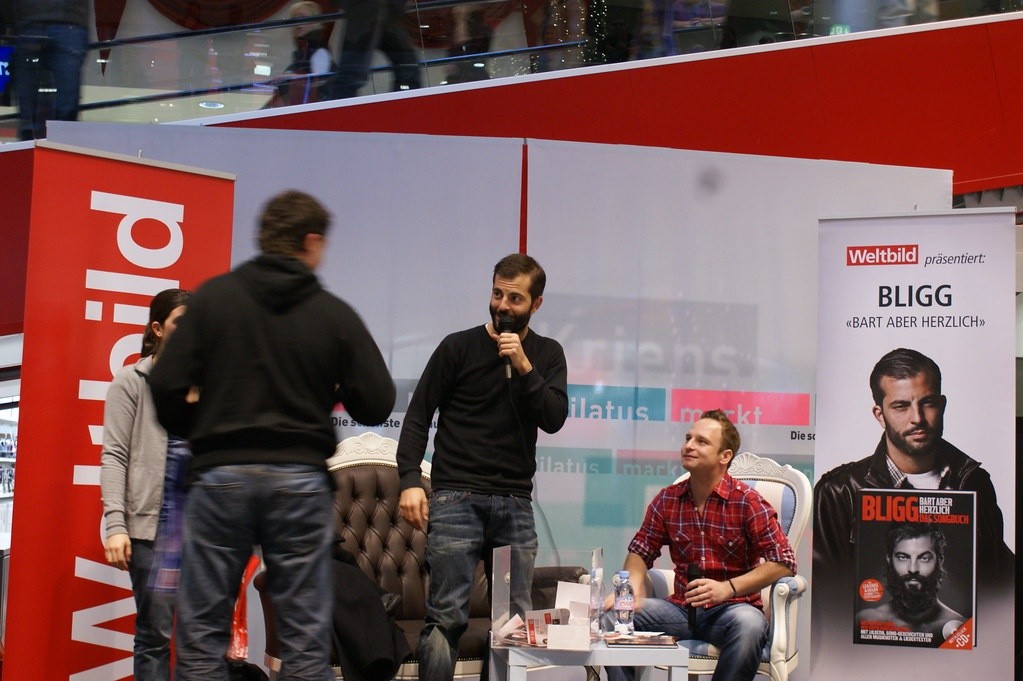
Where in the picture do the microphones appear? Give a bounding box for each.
[686,563,702,628]
[499,317,512,383]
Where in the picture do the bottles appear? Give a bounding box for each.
[612,568,635,636]
[587,569,607,644]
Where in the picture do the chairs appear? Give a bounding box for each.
[303,430,589,680]
[612,452,814,681]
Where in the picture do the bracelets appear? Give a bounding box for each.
[728,579,737,598]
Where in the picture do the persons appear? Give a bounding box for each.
[857,521,963,632]
[446,5,493,84]
[334,0,421,99]
[670,0,725,29]
[101,288,199,681]
[811,348,1015,627]
[273,1,338,108]
[0,0,91,142]
[598,409,797,681]
[145,191,397,681]
[396,253,569,681]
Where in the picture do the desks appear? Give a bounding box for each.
[510,637,688,680]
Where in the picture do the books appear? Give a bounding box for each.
[852,487,978,650]
[604,634,678,649]
